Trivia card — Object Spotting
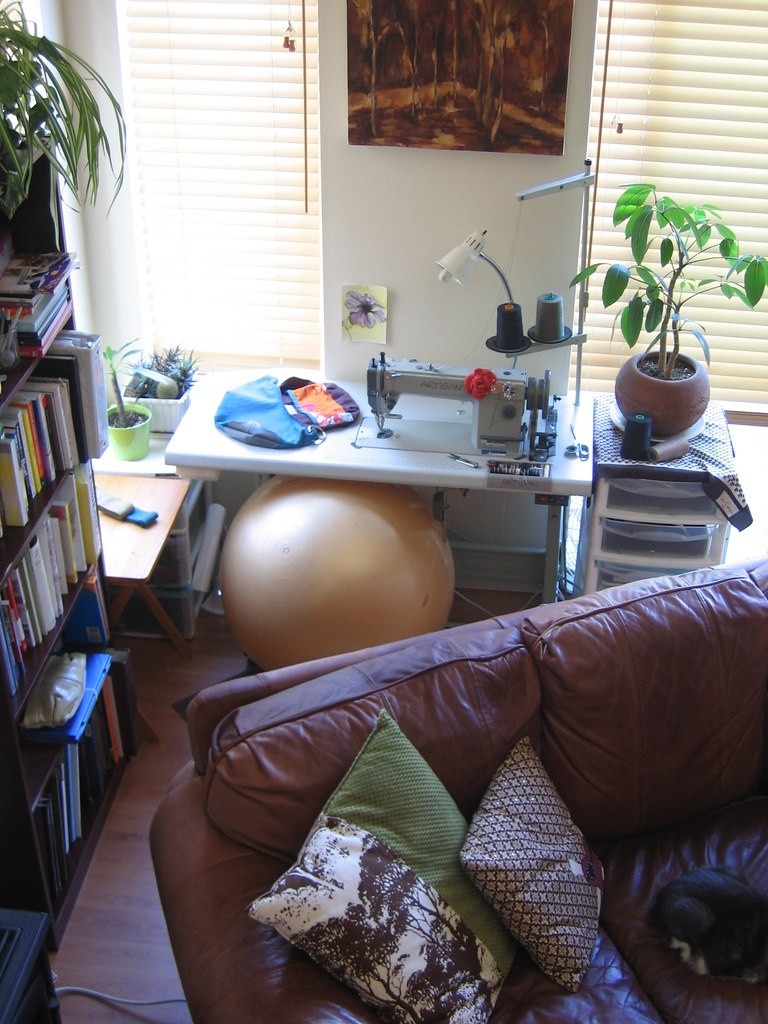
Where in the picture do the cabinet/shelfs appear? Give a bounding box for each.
[572,396,751,597]
[0,138,129,952]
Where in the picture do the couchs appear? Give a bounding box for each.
[149,558,768,1024]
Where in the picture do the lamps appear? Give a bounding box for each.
[434,230,527,350]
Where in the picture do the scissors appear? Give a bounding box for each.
[446,452,479,468]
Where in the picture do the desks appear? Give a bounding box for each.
[165,368,592,605]
[93,476,193,650]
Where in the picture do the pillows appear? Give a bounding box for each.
[243,809,505,1024]
[323,708,518,976]
[460,736,603,994]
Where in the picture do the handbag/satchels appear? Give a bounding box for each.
[215,374,361,450]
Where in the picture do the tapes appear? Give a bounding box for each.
[156,379,178,399]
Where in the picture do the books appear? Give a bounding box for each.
[0,250,83,295]
[0,282,123,912]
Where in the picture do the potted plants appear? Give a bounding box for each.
[102,338,152,460]
[0,2,126,221]
[123,345,198,432]
[569,182,768,437]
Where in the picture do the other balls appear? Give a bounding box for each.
[219,474,458,674]
